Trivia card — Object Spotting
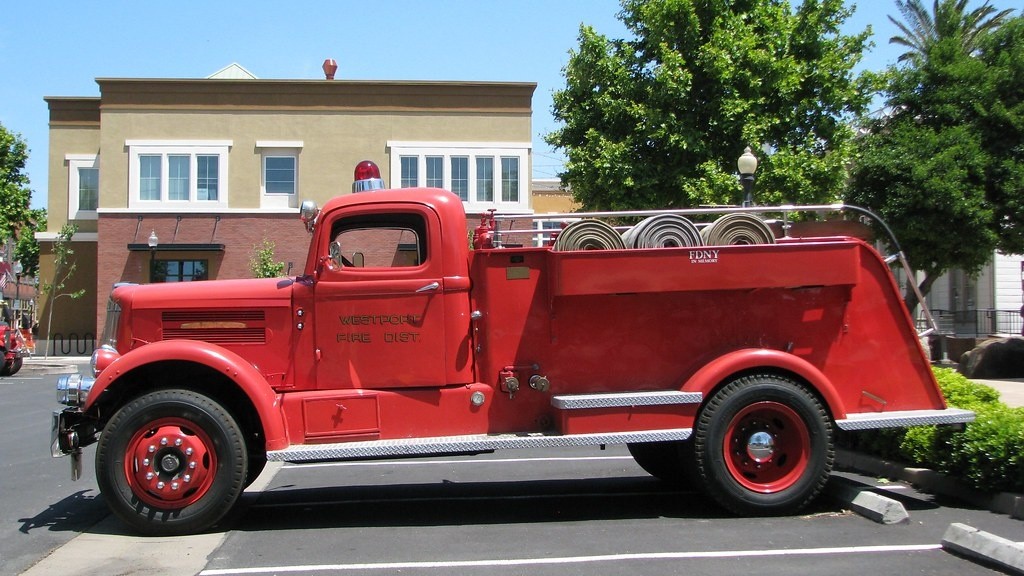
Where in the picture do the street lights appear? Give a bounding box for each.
[738,147,757,207]
[146,229,158,283]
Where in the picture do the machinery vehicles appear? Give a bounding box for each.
[0,300,36,376]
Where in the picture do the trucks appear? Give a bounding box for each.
[47,160,976,538]
